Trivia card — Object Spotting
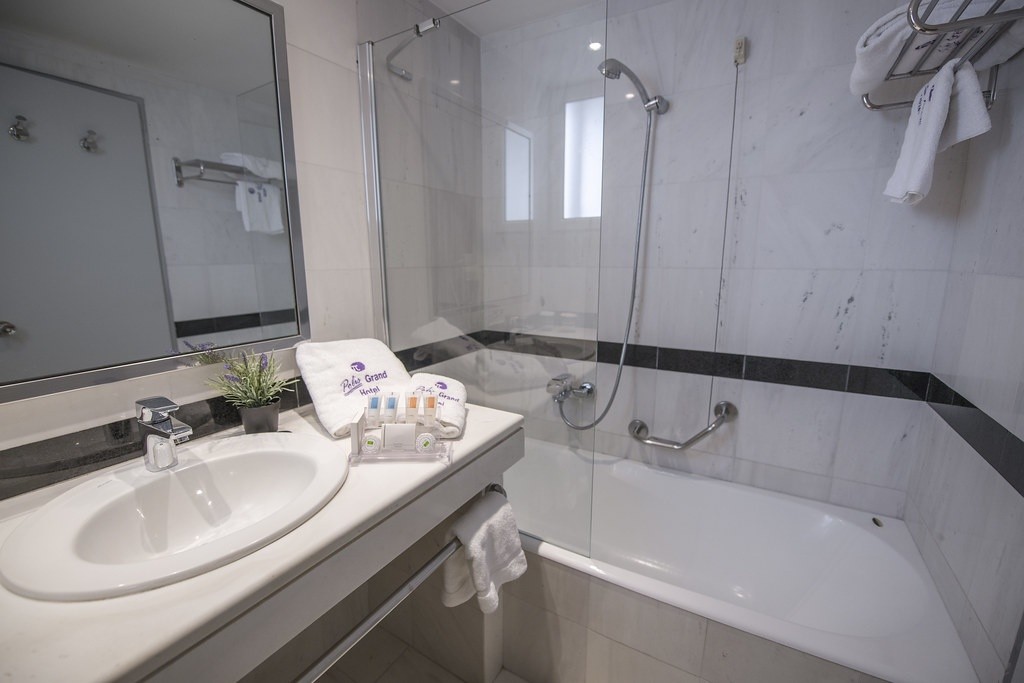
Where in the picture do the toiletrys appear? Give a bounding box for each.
[361,391,439,455]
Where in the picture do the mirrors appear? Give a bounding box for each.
[0,1,313,403]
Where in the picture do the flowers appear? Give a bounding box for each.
[202,350,299,409]
[173,339,218,352]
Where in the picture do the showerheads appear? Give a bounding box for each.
[597,58,650,105]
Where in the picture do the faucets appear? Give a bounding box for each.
[134,395,194,473]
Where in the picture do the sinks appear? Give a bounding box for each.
[72,451,318,565]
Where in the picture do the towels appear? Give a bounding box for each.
[406,317,549,393]
[293,337,468,440]
[440,491,529,615]
[881,57,993,207]
[220,152,283,183]
[235,180,285,236]
[847,0,1024,98]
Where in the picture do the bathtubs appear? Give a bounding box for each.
[502,434,982,683]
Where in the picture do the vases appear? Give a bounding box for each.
[237,397,281,434]
